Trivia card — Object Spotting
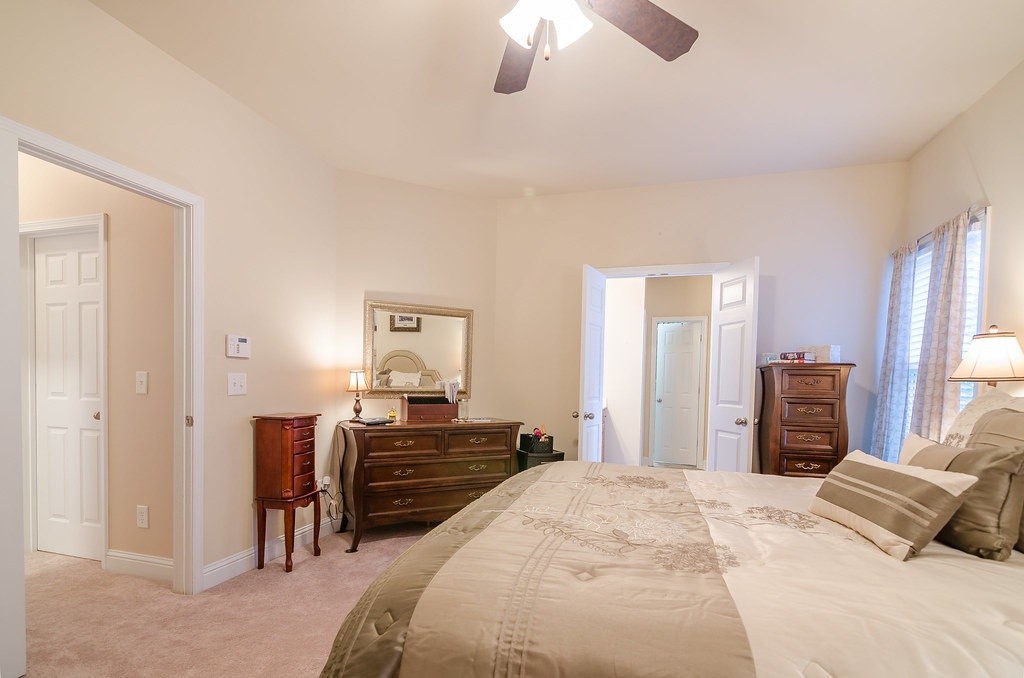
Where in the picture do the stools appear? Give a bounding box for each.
[255,490,323,572]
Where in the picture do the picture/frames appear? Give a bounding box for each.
[390,315,421,332]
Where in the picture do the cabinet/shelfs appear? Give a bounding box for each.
[252,412,322,499]
[515,449,565,473]
[760,361,858,479]
[338,417,525,553]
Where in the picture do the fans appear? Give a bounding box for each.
[492,0,699,94]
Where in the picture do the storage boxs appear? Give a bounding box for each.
[800,344,840,363]
[519,433,553,453]
[402,393,459,422]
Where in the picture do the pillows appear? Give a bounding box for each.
[373,368,421,390]
[809,385,1024,562]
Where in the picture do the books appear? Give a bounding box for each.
[769,359,815,363]
[780,352,816,361]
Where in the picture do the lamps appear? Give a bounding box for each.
[347,370,369,421]
[499,0,594,61]
[947,324,1024,389]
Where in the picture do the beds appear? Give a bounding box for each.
[319,462,1024,678]
[373,349,445,390]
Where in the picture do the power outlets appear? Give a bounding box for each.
[136,505,149,528]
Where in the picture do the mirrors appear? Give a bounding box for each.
[362,299,474,399]
